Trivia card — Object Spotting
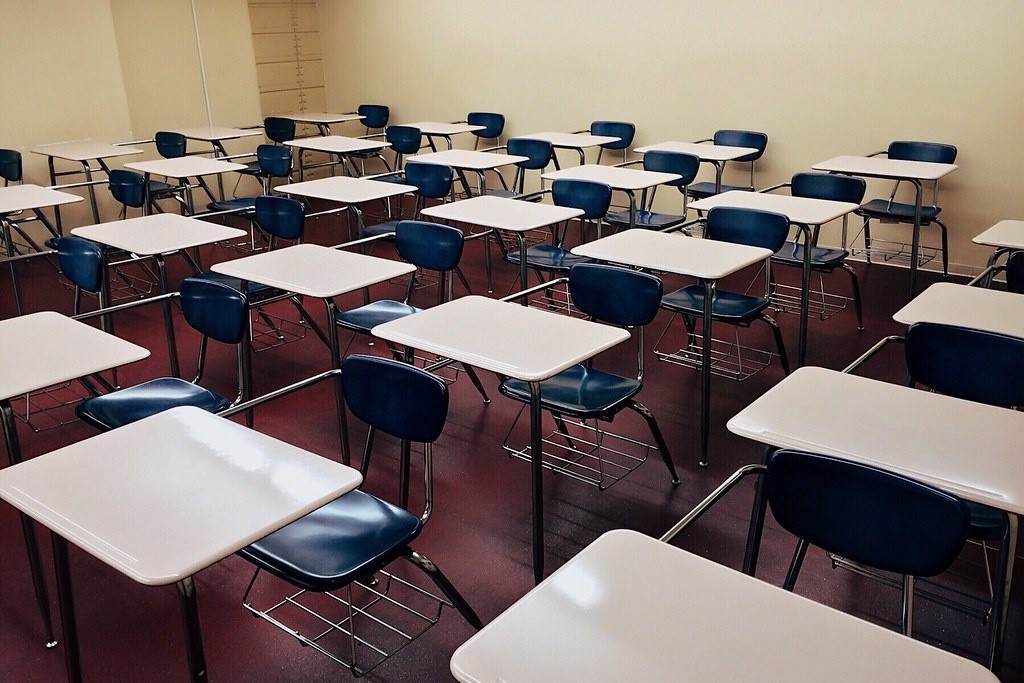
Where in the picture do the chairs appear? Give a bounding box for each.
[0,105,1024,678]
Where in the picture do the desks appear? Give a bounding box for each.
[518,132,622,245]
[29,140,144,299]
[274,112,366,176]
[539,164,683,300]
[891,282,1024,395]
[570,229,773,467]
[371,295,630,586]
[175,126,263,226]
[0,406,363,682]
[726,365,1024,668]
[0,183,85,316]
[812,156,959,299]
[971,220,1024,289]
[632,142,759,195]
[72,211,247,378]
[123,154,249,272]
[421,194,585,307]
[407,147,531,294]
[448,528,1001,683]
[686,190,860,367]
[280,135,392,177]
[274,177,419,346]
[211,244,417,467]
[0,311,151,648]
[396,122,487,228]
